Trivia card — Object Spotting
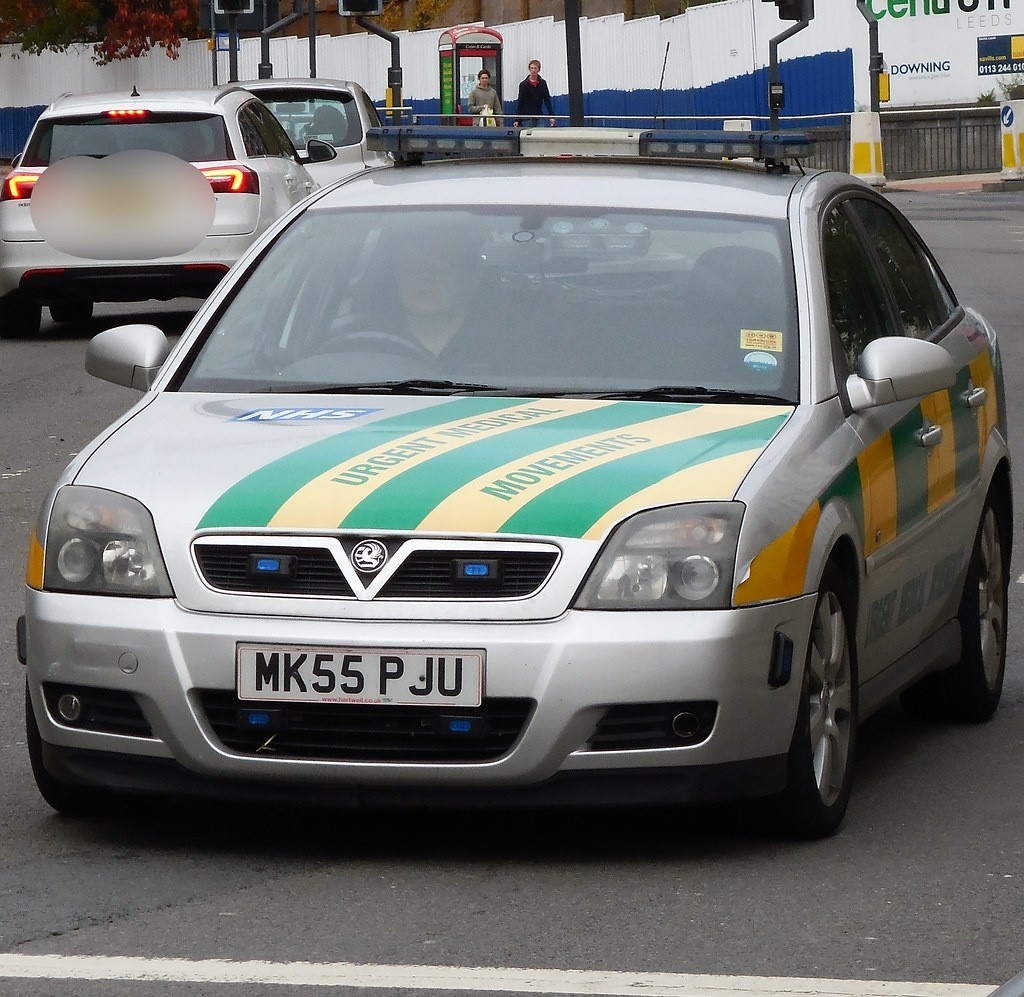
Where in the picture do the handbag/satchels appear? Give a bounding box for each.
[479,108,496,127]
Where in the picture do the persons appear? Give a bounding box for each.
[306,224,501,376]
[513,60,556,128]
[468,69,503,127]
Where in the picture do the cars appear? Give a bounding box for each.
[16,157,1013,854]
[222,77,394,193]
[1,86,317,329]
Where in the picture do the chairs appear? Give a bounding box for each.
[312,105,348,143]
[673,244,788,393]
[81,124,118,158]
[166,123,208,158]
